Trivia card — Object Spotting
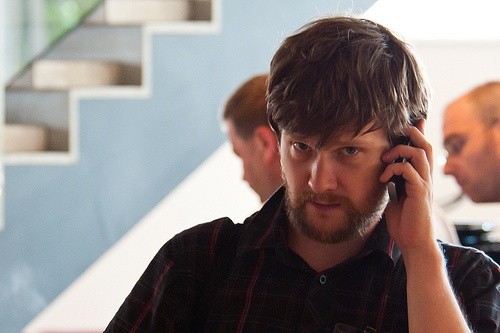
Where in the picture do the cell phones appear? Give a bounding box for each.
[387,136,415,203]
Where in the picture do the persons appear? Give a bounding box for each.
[103,17,500,333]
[440,81,500,203]
[223,74,287,204]
